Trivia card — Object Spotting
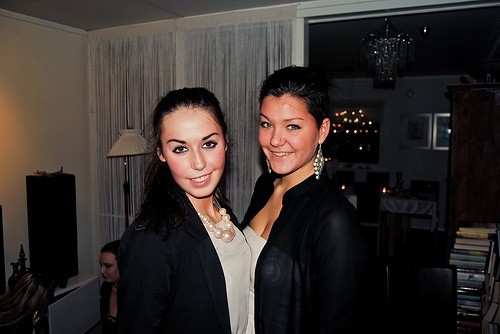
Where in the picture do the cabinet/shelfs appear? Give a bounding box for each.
[443,83,500,243]
[47,272,101,334]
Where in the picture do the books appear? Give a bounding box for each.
[449,223,500,334]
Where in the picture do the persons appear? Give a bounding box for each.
[98,240,121,334]
[239,66,362,334]
[0,271,55,334]
[116,87,252,334]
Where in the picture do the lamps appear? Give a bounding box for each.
[361,18,429,91]
[106,126,155,228]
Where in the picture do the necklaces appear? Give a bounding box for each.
[197,208,236,243]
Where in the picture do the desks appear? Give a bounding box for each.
[379,192,438,256]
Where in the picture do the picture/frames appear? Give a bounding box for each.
[433,112,451,152]
[399,113,432,151]
